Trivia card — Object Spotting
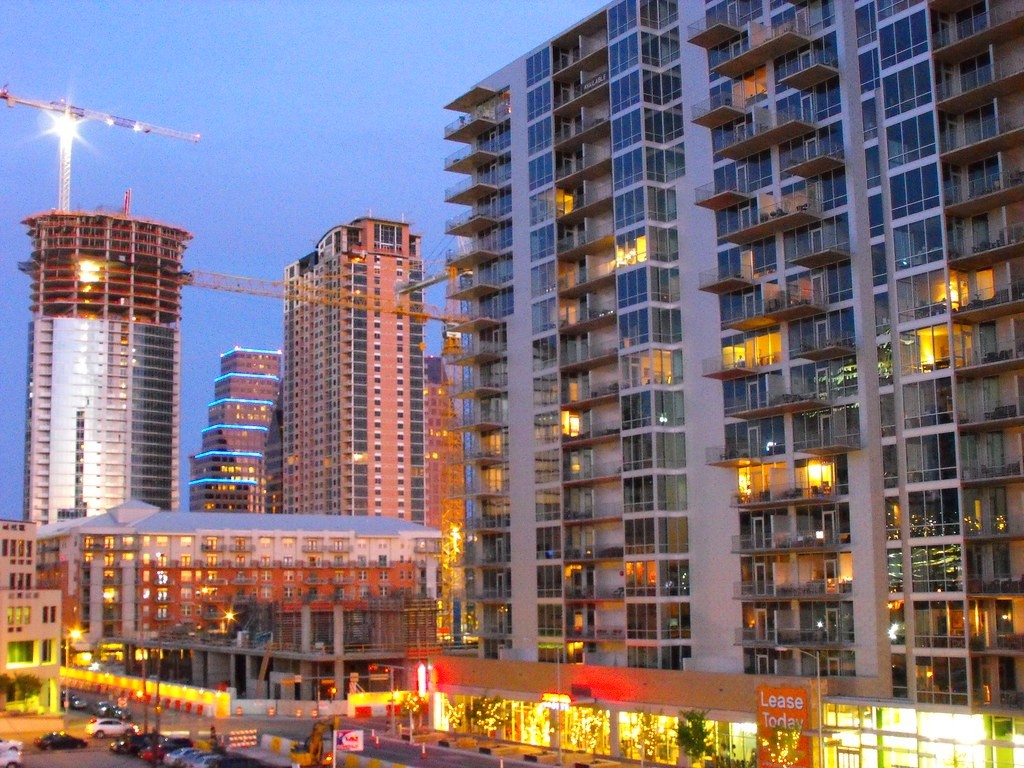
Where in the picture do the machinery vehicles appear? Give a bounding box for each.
[287,716,340,768]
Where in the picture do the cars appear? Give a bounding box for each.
[34,732,88,750]
[0,738,23,752]
[1,750,22,768]
[88,717,140,738]
[62,691,133,720]
[111,731,274,768]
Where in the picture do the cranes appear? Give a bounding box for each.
[183,254,470,641]
[0,85,200,215]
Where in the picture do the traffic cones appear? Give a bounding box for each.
[421,742,427,760]
[374,736,380,749]
[370,728,376,741]
[498,754,506,768]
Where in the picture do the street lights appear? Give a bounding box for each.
[63,628,82,716]
[773,643,829,768]
